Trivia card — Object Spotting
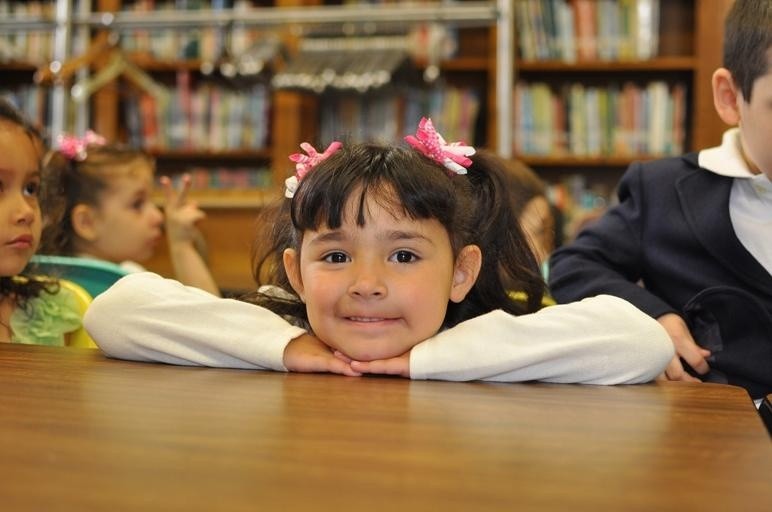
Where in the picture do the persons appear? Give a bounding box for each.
[36,126,223,299]
[551,0,772,398]
[0,91,84,348]
[84,114,678,385]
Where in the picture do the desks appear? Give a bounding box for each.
[0,339,772,511]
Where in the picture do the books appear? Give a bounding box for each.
[0,1,689,287]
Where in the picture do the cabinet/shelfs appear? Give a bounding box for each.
[0,0,738,288]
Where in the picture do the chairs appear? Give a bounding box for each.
[29,253,125,297]
